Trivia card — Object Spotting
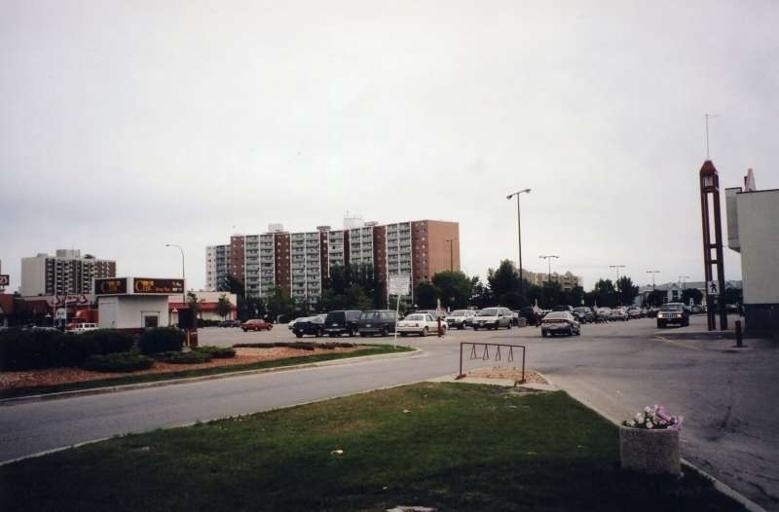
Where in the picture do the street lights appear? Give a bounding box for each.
[645,269,662,291]
[608,264,627,292]
[539,253,561,282]
[678,275,691,289]
[505,188,536,296]
[445,237,458,275]
[165,241,187,281]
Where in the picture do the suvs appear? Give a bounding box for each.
[359,309,404,337]
[657,299,691,330]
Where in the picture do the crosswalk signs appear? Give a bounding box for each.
[706,280,721,298]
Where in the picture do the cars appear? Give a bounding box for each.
[540,309,581,336]
[286,319,296,330]
[217,319,240,328]
[688,303,737,313]
[292,313,326,338]
[413,304,657,332]
[240,318,273,331]
[395,312,449,337]
[2,321,99,335]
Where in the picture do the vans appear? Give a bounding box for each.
[324,308,363,338]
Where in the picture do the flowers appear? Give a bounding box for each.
[617,400,684,429]
